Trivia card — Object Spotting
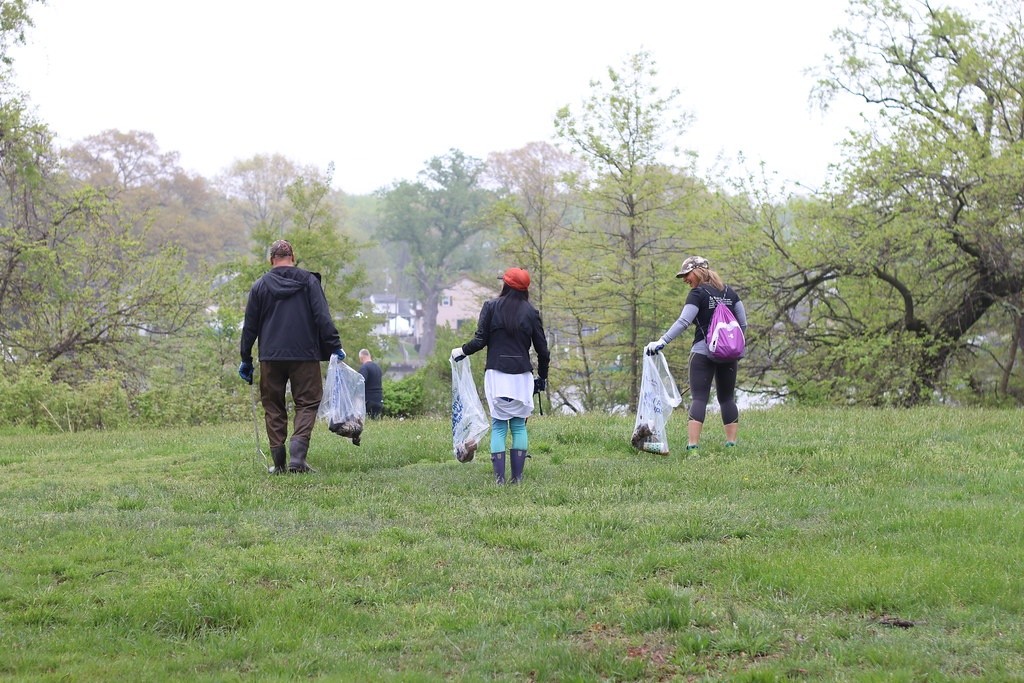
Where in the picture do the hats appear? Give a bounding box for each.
[497,268,530,291]
[270,239,293,255]
[676,256,710,278]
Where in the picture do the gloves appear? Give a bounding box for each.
[534,374,546,393]
[335,349,347,361]
[646,339,666,357]
[238,361,255,386]
[451,347,467,361]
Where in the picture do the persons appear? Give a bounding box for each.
[238,239,345,475]
[451,267,550,485]
[645,255,747,460]
[357,348,383,422]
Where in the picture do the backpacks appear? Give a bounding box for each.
[695,283,746,362]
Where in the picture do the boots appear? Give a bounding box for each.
[491,451,506,484]
[268,445,287,474]
[288,436,315,473]
[509,448,526,484]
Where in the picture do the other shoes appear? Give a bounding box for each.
[686,445,699,454]
[725,442,736,447]
